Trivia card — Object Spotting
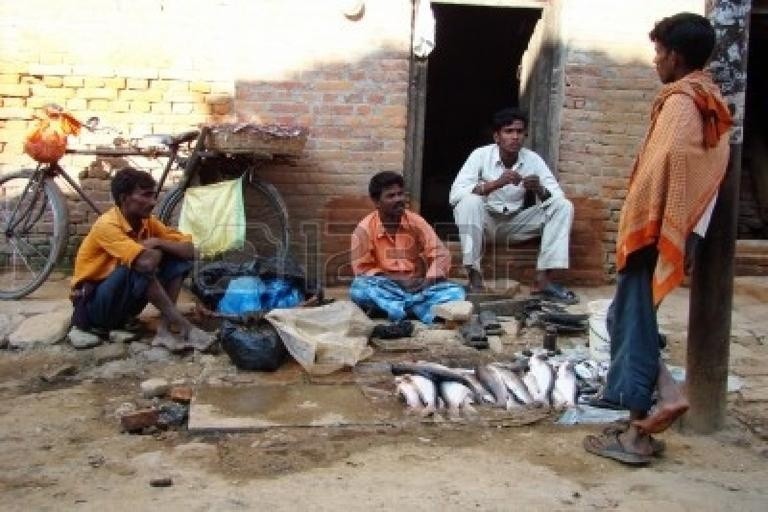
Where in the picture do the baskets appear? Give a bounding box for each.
[203,119,311,157]
[192,290,321,329]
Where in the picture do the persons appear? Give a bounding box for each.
[68,167,222,353]
[449,111,581,305]
[585,13,731,467]
[350,171,466,329]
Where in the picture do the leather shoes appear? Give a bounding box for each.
[530,280,580,305]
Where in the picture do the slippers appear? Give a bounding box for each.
[583,431,652,465]
[476,308,503,336]
[456,322,488,347]
[603,419,665,455]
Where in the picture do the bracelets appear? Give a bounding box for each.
[480,181,487,195]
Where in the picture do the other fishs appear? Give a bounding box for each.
[390,347,610,423]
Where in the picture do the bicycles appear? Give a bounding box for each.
[0,105,291,301]
